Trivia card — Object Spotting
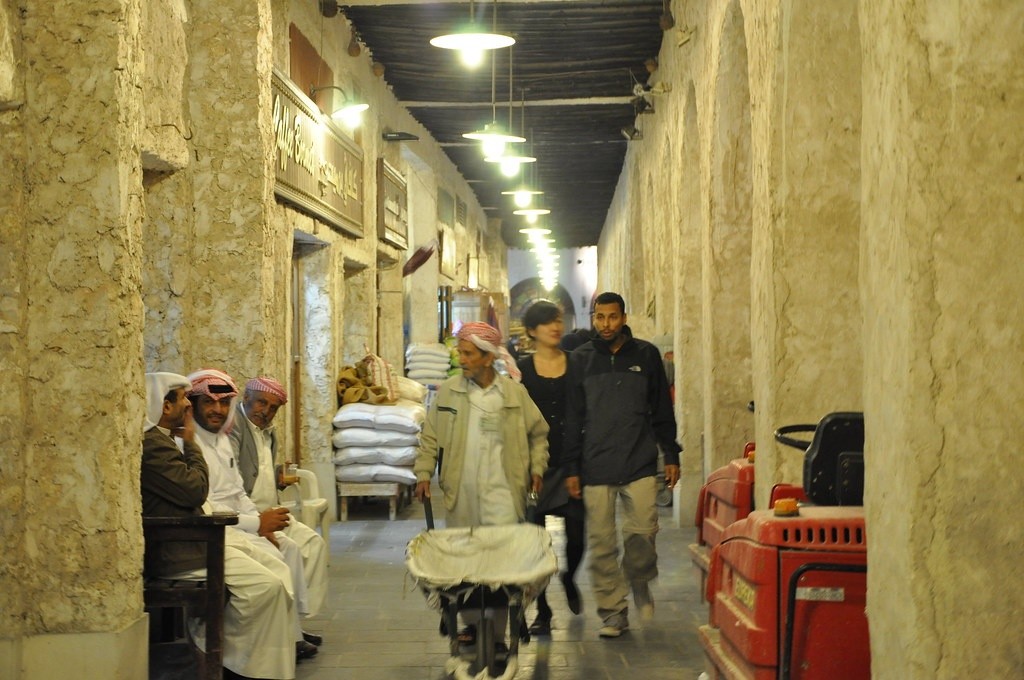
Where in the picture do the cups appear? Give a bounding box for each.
[283,463,299,484]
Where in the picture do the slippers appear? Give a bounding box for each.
[493,643,507,674]
[457,624,479,645]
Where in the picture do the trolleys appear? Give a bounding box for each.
[421,490,559,679]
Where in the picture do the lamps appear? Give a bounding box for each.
[429,0,560,292]
[621,124,642,139]
[310,86,369,132]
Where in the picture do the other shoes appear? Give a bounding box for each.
[300,631,322,646]
[531,610,552,635]
[294,642,318,660]
[631,582,656,621]
[561,573,581,615]
[600,624,627,637]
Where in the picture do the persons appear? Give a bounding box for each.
[140,372,296,680]
[175,368,327,659]
[517,301,585,632]
[560,329,588,351]
[562,292,680,637]
[415,322,550,657]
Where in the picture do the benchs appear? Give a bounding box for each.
[142,512,234,680]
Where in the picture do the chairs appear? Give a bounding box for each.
[279,468,330,568]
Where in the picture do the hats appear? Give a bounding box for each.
[247,378,288,405]
[458,321,521,383]
